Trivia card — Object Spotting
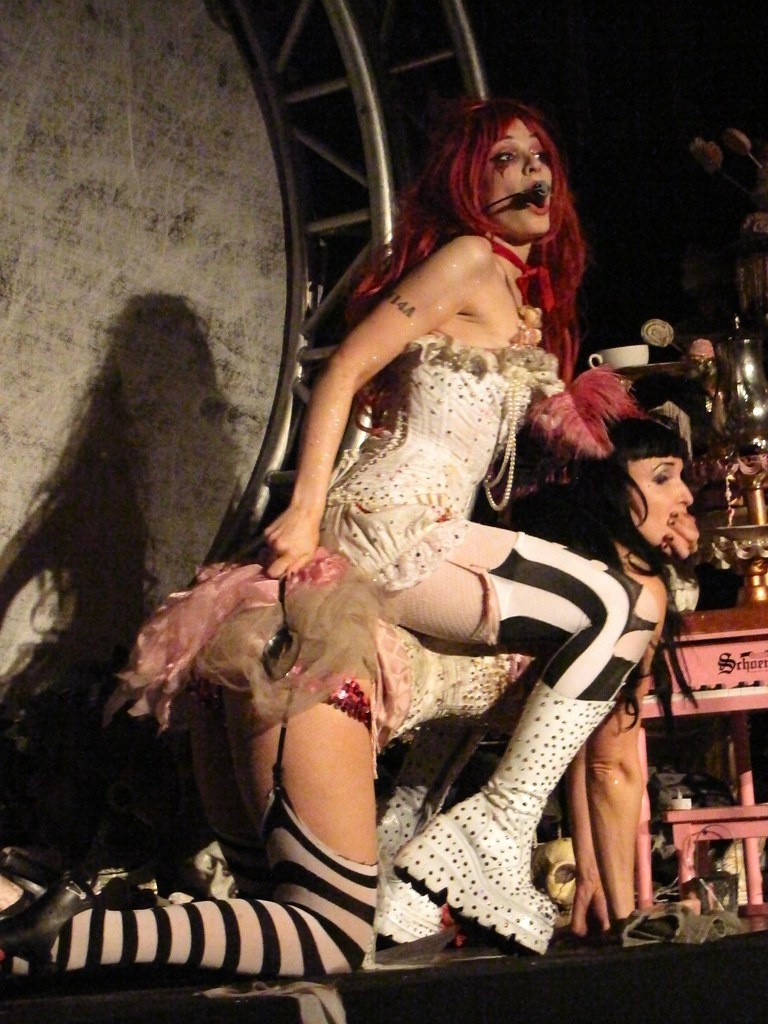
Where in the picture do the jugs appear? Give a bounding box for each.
[707,313,768,439]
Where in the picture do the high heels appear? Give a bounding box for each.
[0,845,103,985]
[0,847,56,925]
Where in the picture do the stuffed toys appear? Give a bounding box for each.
[512,304,543,346]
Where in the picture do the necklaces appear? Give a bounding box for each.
[484,235,556,314]
[496,253,543,283]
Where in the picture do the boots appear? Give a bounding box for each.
[388,682,621,961]
[377,720,486,943]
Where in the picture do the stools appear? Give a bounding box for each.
[662,806,768,918]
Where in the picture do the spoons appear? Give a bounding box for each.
[263,578,300,681]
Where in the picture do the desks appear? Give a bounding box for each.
[615,601,768,909]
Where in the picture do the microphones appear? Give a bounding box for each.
[481,181,550,214]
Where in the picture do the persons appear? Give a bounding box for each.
[264,97,700,982]
[0,418,703,978]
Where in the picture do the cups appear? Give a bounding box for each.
[589,344,649,369]
